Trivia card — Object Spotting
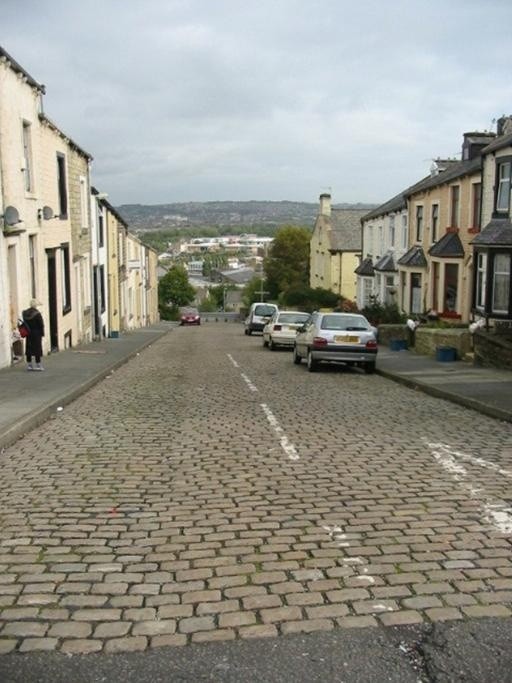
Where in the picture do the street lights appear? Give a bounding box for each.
[256,261,263,302]
[223,287,227,312]
[93,192,109,341]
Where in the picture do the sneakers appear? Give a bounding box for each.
[26,362,33,371]
[35,362,44,371]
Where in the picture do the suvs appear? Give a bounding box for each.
[179,307,202,325]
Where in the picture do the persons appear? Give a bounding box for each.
[22,298,45,372]
[407,315,418,348]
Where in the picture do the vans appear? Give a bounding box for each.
[245,302,279,335]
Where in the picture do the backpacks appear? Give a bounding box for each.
[18,309,39,338]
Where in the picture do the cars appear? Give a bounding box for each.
[261,310,311,350]
[293,310,378,373]
